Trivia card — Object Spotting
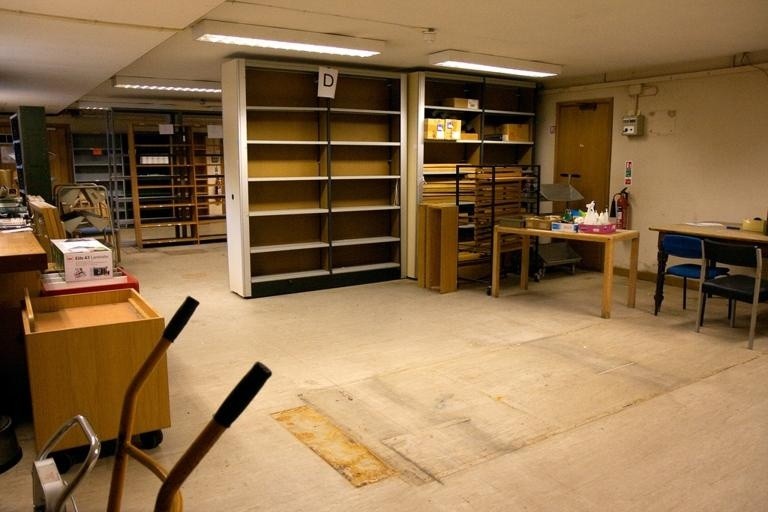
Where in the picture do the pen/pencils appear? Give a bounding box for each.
[726,227,740,229]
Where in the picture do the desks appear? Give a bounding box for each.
[491,217,640,318]
[648,221,768,317]
[0,228,46,426]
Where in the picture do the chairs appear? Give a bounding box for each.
[694,238,768,349]
[652,233,732,319]
[56,185,121,262]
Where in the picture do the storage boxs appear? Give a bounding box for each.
[445,119,462,141]
[496,124,533,142]
[49,237,114,282]
[424,118,445,140]
[442,97,480,110]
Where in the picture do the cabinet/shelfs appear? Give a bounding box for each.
[220,58,409,296]
[18,287,170,473]
[42,264,139,296]
[408,67,540,294]
[0,106,226,250]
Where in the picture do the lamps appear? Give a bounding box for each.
[190,19,386,58]
[427,48,563,80]
[111,76,222,93]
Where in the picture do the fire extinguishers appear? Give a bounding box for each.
[609,187,629,230]
